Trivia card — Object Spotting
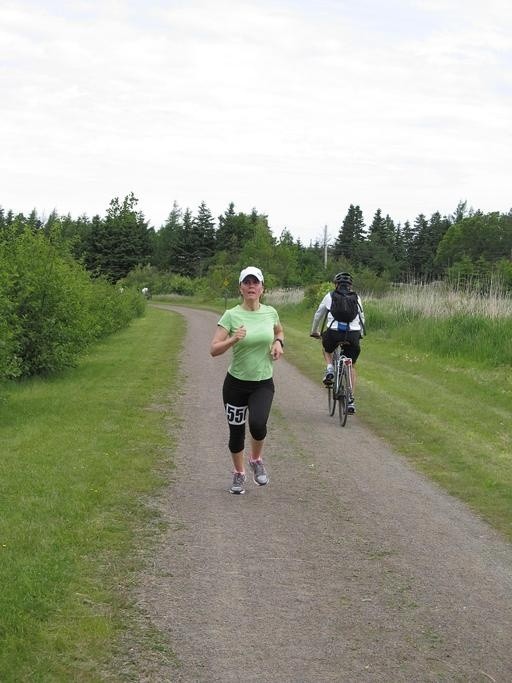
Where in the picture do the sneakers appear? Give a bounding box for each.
[323,371,334,385]
[249,455,269,486]
[348,403,356,413]
[229,468,246,495]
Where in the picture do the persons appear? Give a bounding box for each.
[312,271,366,412]
[210,265,285,493]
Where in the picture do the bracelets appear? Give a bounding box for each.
[275,338,283,347]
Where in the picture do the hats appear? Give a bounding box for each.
[238,266,264,285]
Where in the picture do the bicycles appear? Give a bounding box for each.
[310,334,354,427]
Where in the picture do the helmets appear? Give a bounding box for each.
[334,272,353,285]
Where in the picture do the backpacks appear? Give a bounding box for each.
[330,290,358,323]
